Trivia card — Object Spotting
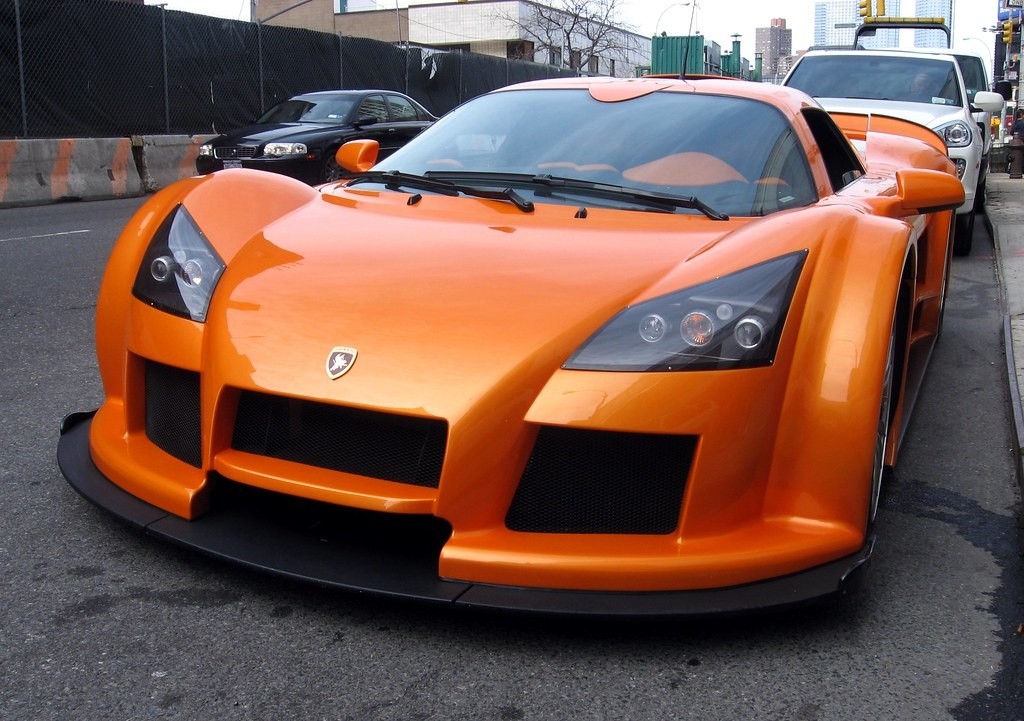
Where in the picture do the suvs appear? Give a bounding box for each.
[864,45,1014,215]
[778,47,1006,258]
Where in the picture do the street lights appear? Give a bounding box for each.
[654,2,691,37]
[963,36,993,90]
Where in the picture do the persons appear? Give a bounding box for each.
[1010,109,1024,138]
[910,74,944,96]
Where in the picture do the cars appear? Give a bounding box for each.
[194,88,440,188]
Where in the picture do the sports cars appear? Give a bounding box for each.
[56,74,969,621]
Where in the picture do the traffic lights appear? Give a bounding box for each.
[859,0,872,17]
[1001,21,1013,45]
